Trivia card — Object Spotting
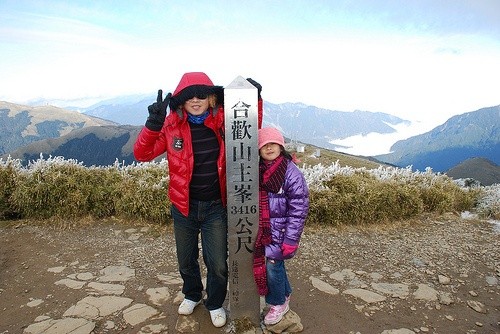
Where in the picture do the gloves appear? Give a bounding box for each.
[246,78,262,100]
[146,90,171,131]
[282,243,298,259]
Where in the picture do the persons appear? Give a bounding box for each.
[258,127,308,325]
[134,72,263,327]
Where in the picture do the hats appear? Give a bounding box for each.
[258,126,284,150]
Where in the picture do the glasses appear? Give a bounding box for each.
[185,92,207,100]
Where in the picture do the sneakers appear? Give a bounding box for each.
[265,302,289,324]
[285,294,291,305]
[178,299,201,315]
[210,307,226,327]
